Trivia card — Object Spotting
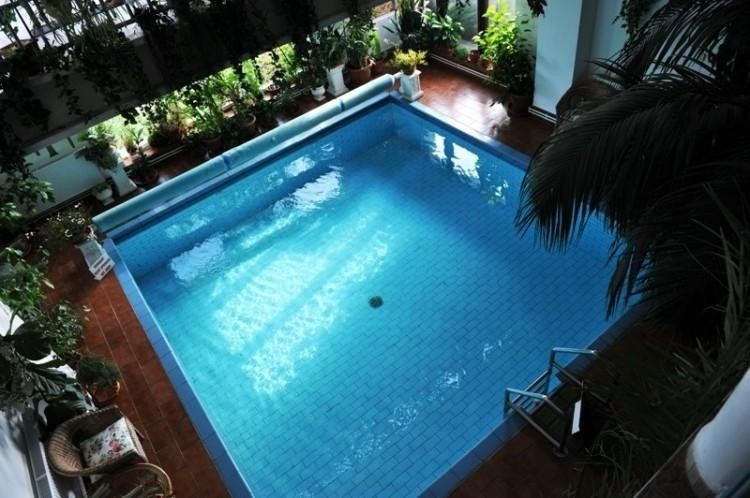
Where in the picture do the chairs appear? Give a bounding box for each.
[45,403,151,479]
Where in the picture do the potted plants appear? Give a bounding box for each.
[77,355,121,407]
[291,26,327,101]
[73,124,137,198]
[163,68,224,156]
[393,49,429,102]
[371,35,385,74]
[40,210,95,250]
[317,25,350,98]
[344,4,373,84]
[470,6,537,117]
[209,59,256,138]
